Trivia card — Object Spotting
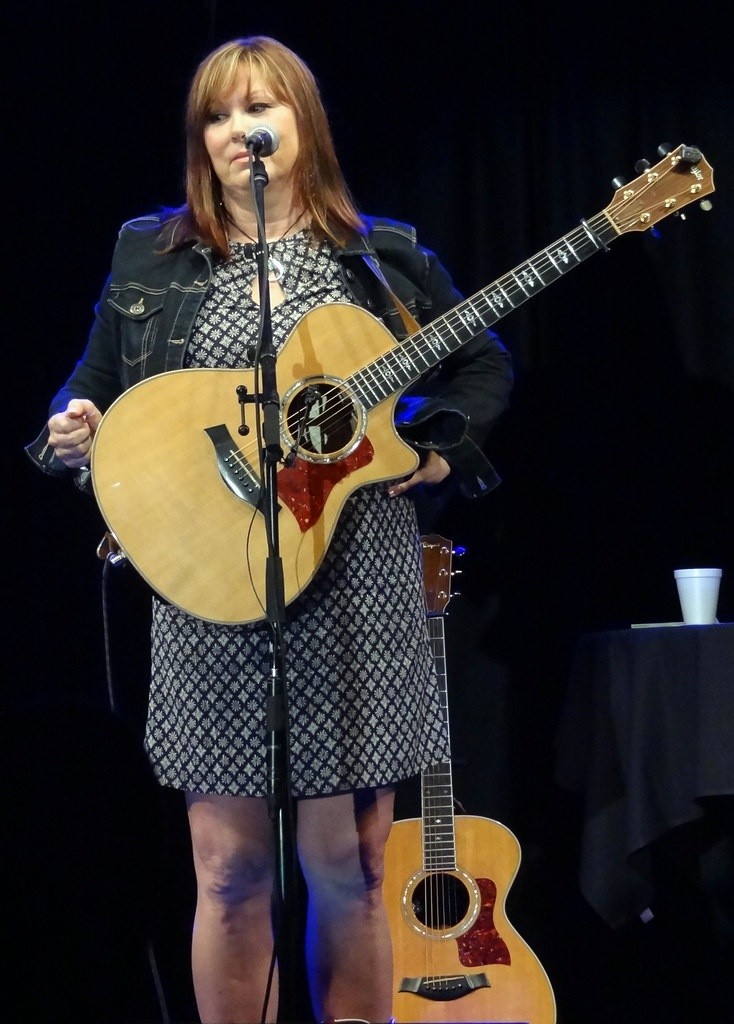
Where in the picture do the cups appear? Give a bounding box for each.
[675,569,723,625]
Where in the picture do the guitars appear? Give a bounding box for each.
[382,532,560,1024]
[89,142,703,627]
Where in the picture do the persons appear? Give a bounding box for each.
[23,34,514,1023]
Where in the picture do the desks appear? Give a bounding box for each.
[580,624,734,931]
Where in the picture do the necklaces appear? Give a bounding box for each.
[226,208,309,310]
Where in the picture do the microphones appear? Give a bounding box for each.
[246,125,280,157]
